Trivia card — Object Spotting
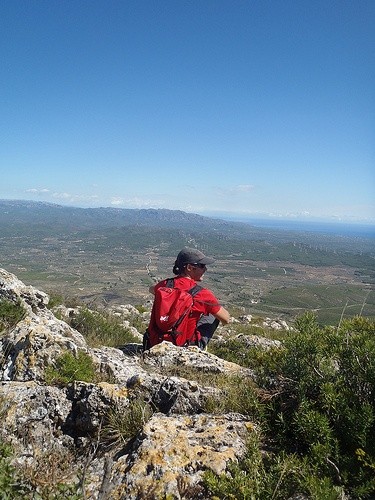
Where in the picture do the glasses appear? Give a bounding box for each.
[186,264,205,271]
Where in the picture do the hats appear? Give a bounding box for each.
[176,248,216,265]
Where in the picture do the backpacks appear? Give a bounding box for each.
[146,277,203,347]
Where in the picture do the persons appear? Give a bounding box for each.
[142,248,230,352]
